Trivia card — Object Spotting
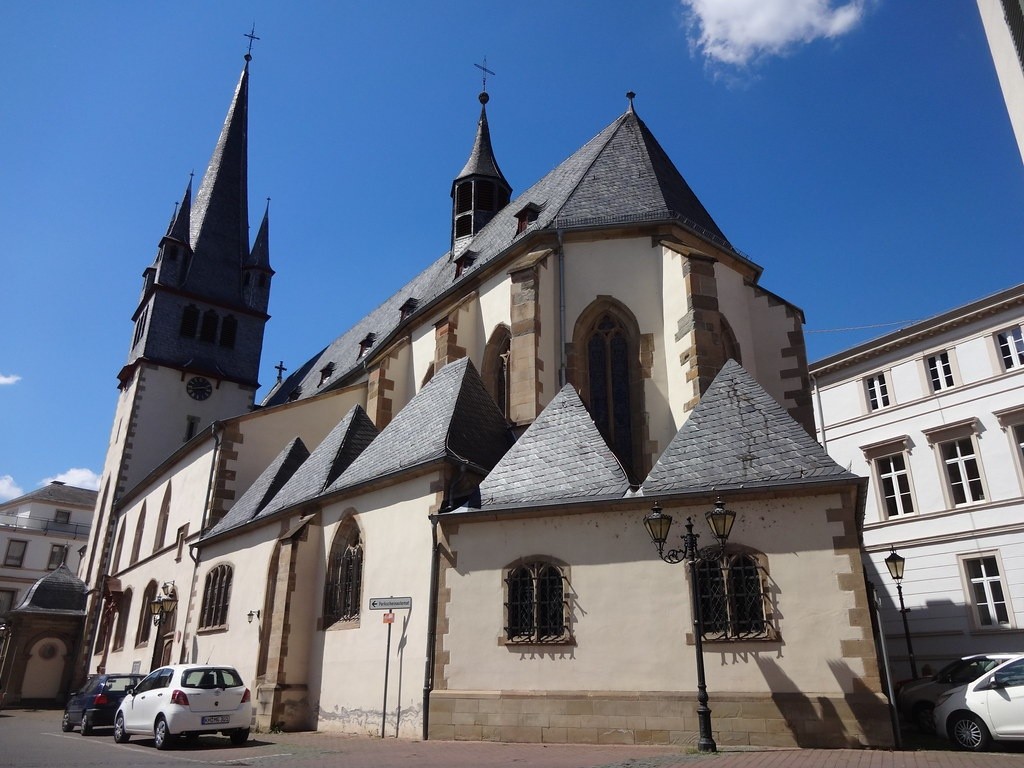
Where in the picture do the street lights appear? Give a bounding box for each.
[643,496,737,756]
[884,543,920,678]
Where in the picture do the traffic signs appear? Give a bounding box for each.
[369,596,413,610]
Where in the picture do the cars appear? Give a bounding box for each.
[931,654,1024,753]
[897,652,1023,735]
[62,673,152,736]
[113,663,253,751]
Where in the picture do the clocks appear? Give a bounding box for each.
[186,377,213,401]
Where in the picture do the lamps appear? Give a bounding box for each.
[162,580,175,595]
[248,610,261,623]
